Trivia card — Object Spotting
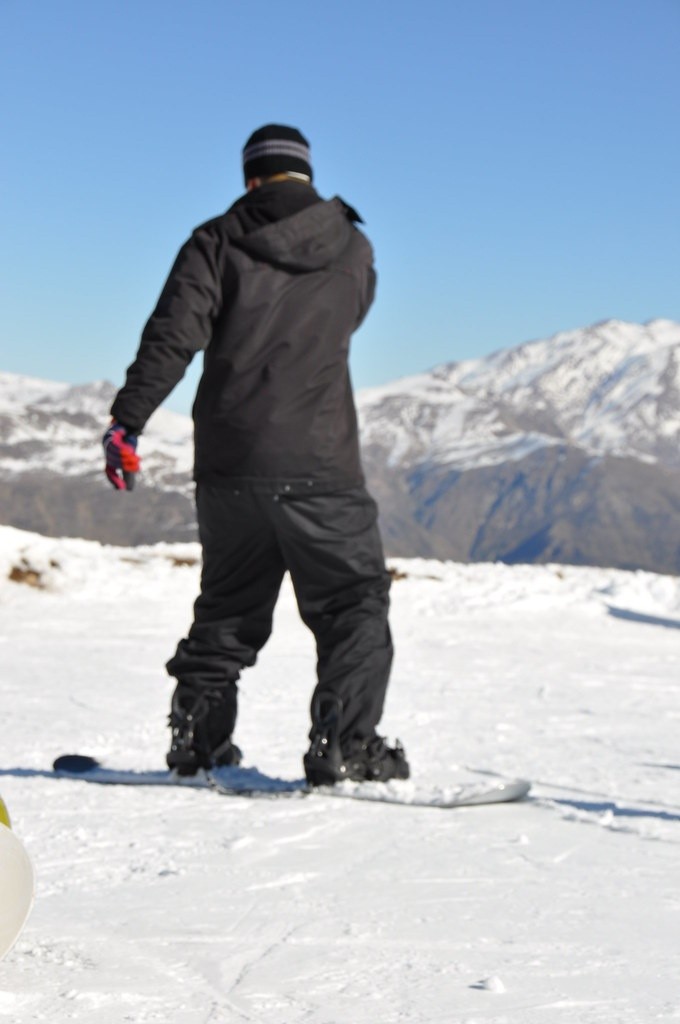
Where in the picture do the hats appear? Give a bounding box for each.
[242,123,314,185]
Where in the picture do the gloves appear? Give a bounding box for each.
[101,417,141,492]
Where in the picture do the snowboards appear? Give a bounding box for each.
[52,751,535,810]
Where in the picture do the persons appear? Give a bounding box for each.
[102,124,411,787]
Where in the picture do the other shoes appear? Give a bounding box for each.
[166,683,243,776]
[304,691,411,785]
